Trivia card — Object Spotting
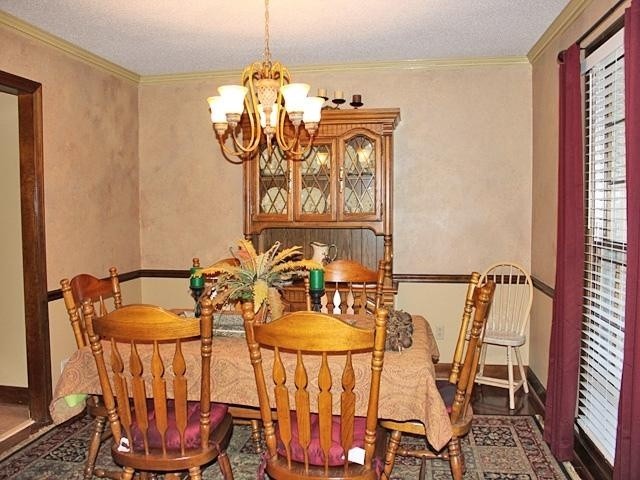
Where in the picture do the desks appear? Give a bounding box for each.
[62,308,451,452]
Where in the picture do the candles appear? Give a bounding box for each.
[317,88,327,97]
[353,94,361,103]
[334,90,344,98]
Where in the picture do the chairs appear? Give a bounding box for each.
[466,261,534,411]
[379,271,496,477]
[192,259,244,314]
[302,259,384,314]
[59,266,165,479]
[241,300,386,479]
[79,296,237,479]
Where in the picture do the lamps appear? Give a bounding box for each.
[206,0,325,165]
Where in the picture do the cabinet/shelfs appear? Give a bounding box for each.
[241,106,401,235]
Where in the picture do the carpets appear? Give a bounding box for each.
[0,413,569,480]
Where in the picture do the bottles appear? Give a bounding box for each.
[309,241,338,266]
